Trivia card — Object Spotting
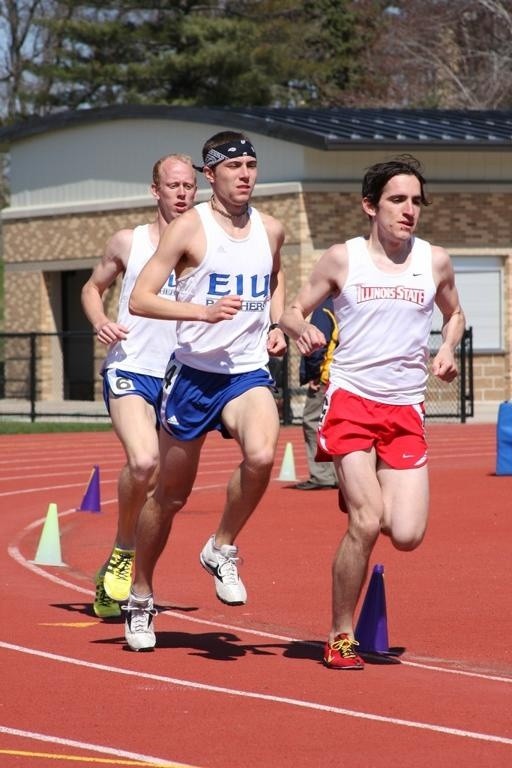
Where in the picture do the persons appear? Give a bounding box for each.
[80,153,198,617]
[121,130,288,652]
[279,154,466,669]
[296,295,340,490]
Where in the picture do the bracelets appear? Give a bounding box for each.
[269,324,286,336]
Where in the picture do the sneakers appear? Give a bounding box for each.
[323,632,366,670]
[199,535,248,606]
[124,588,157,652]
[92,566,121,618]
[104,546,135,602]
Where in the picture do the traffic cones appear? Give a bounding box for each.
[270,436,302,488]
[23,497,67,573]
[72,465,110,518]
[343,560,406,661]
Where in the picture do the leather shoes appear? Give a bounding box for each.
[295,481,338,490]
